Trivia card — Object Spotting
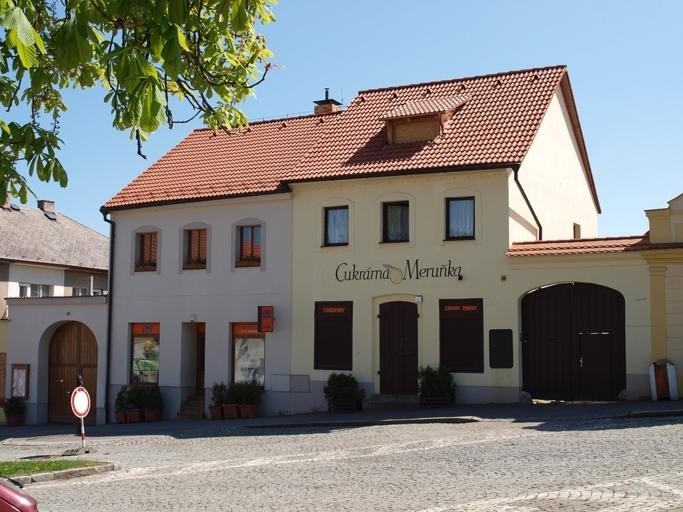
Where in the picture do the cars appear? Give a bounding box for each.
[0,472,38,511]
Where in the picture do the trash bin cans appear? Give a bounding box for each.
[649,359,679,401]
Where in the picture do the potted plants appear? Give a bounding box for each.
[417,363,455,405]
[3,398,26,426]
[208,381,261,417]
[324,372,362,413]
[114,384,163,422]
